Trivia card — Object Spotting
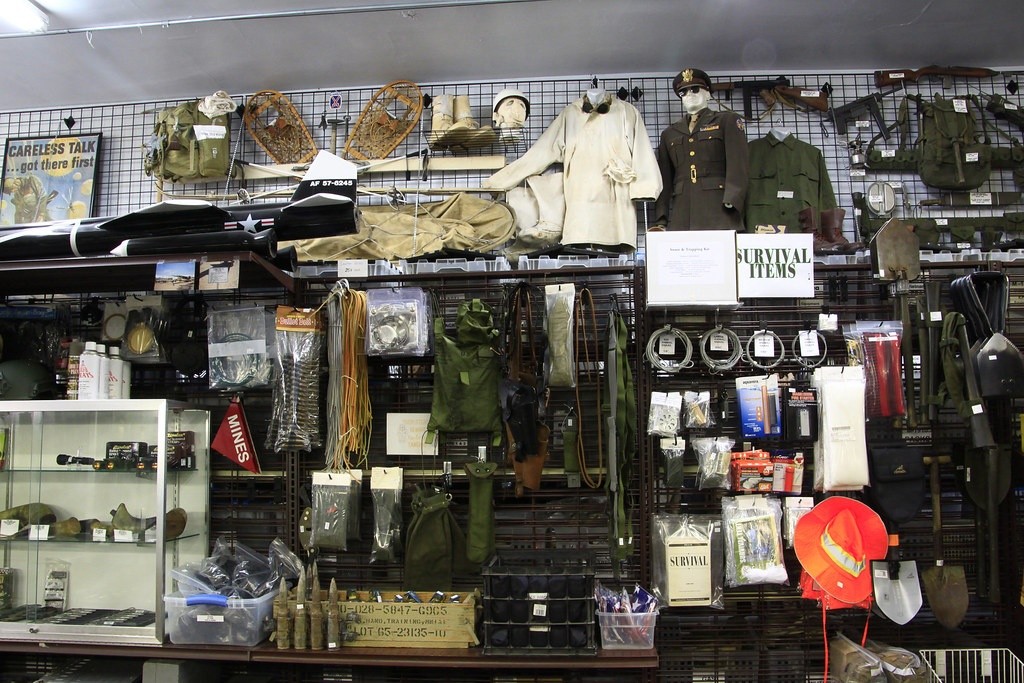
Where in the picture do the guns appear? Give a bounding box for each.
[710,76,829,118]
[828,86,904,141]
[874,65,1024,89]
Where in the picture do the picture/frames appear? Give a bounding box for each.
[0,132,103,231]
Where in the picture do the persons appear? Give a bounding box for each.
[490,89,530,141]
[486,67,840,252]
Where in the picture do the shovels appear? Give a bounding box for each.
[866,440,925,626]
[949,269,1024,401]
[965,440,1014,611]
[869,217,922,431]
[921,453,971,631]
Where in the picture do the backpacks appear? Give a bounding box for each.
[145,102,232,183]
[869,94,994,191]
[424,298,503,446]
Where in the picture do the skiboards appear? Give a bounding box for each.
[154,155,509,198]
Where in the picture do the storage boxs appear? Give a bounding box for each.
[645,230,739,311]
[480,549,598,658]
[274,589,481,649]
[163,581,293,646]
[595,609,660,650]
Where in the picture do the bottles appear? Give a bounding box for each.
[96,344,109,399]
[110,347,123,397]
[79,341,100,399]
[123,362,131,399]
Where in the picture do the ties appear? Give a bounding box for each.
[689,114,700,134]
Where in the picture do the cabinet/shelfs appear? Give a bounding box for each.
[0,69,1024,683]
[0,399,214,644]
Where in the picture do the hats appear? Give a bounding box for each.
[672,68,711,98]
[795,497,889,604]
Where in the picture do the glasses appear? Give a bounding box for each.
[679,86,699,96]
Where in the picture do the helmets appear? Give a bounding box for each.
[493,89,530,123]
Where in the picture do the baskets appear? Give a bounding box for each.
[919,648,1024,683]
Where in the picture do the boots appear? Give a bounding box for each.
[430,94,495,150]
[799,206,865,251]
